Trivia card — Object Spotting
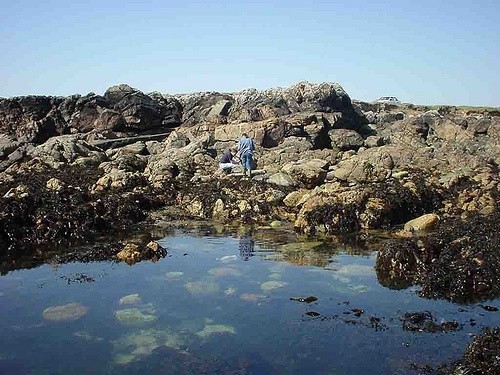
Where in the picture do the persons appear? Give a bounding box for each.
[219,147,241,174]
[238,133,255,177]
